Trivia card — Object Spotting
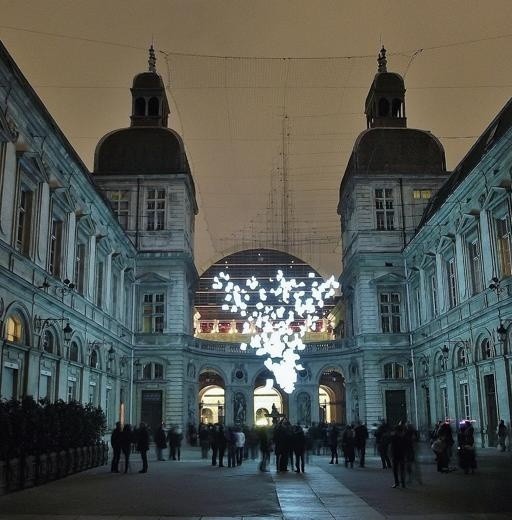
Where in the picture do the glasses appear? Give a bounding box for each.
[35,316,73,335]
[87,341,115,363]
[496,318,512,341]
[442,339,469,359]
[120,356,146,366]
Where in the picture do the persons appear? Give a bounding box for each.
[496,419,507,451]
